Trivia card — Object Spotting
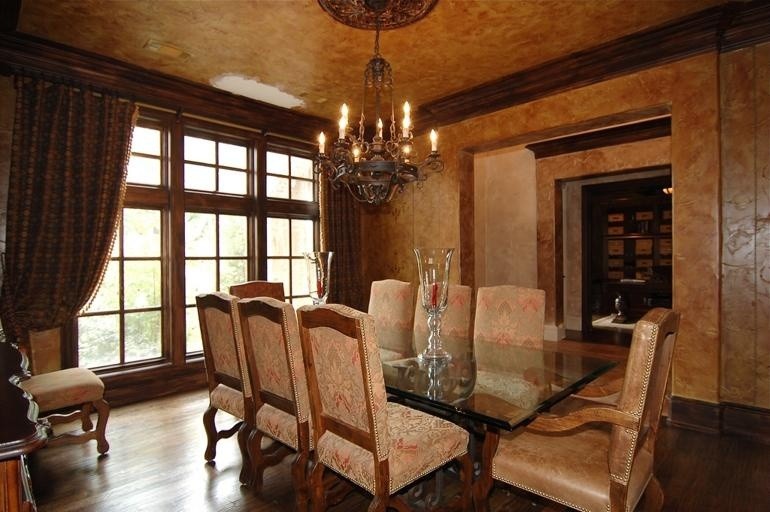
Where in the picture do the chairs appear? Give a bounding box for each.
[246,296,317,509]
[440,283,549,427]
[194,291,262,487]
[382,280,475,387]
[294,304,474,510]
[488,306,679,511]
[13,366,113,454]
[362,278,415,363]
[229,280,286,303]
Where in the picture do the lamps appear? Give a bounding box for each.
[661,175,672,196]
[295,0,447,206]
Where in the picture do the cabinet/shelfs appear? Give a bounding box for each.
[597,196,672,318]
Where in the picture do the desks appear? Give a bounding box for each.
[0,342,50,512]
[369,326,623,512]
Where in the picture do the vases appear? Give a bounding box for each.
[412,245,456,363]
[302,250,334,307]
